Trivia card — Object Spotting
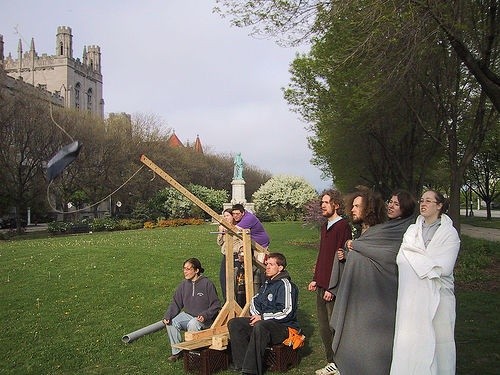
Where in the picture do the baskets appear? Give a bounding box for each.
[262,345,300,372]
[184,351,229,375]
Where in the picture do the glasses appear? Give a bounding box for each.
[417,200,438,204]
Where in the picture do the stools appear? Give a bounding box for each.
[184,345,229,375]
[264,344,300,373]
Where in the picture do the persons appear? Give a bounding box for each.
[389,190,461,375]
[232,152,244,179]
[162,258,221,361]
[308,190,352,375]
[216,204,271,307]
[227,253,299,375]
[327,191,420,375]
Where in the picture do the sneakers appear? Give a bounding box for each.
[168,352,183,361]
[315,363,338,375]
